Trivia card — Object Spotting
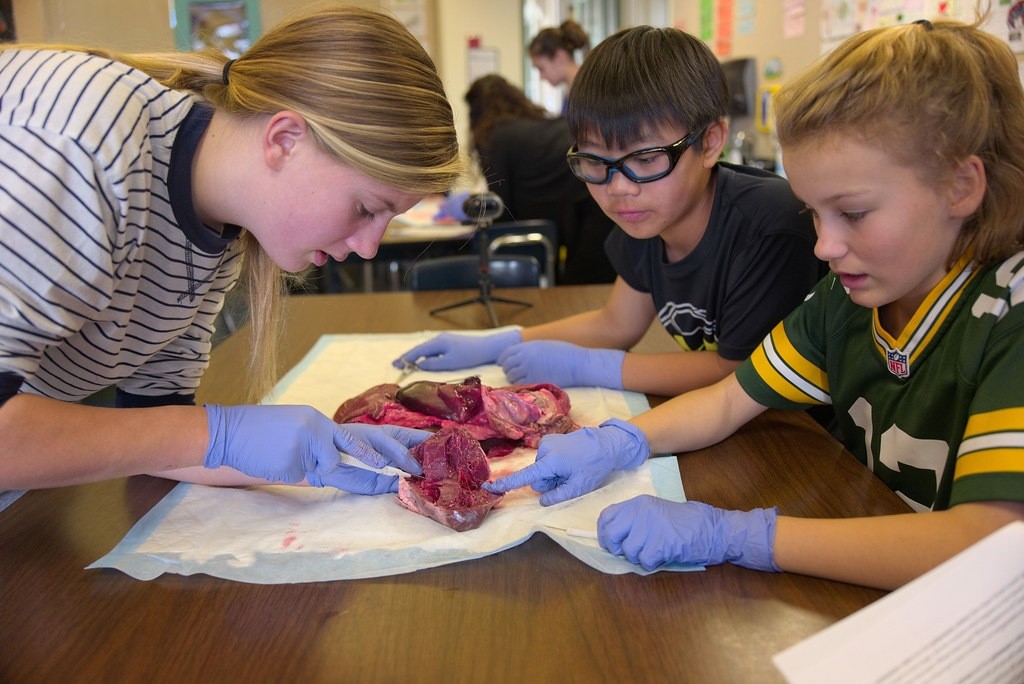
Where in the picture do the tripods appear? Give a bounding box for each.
[429,222,534,329]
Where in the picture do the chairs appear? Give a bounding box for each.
[409,253,542,286]
[479,219,559,285]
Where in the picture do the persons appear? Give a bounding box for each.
[432,73,618,286]
[527,17,589,117]
[479,19,1024,591]
[391,24,830,395]
[0,5,460,493]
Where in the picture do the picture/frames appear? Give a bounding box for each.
[465,48,500,87]
[755,83,782,134]
[175,0,263,60]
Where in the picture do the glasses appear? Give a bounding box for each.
[559,131,695,184]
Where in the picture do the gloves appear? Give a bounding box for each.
[596,492,781,574]
[201,400,384,484]
[394,328,524,371]
[434,192,477,223]
[497,338,627,393]
[306,420,437,494]
[481,418,651,507]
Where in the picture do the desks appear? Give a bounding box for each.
[0,285,1024,684]
